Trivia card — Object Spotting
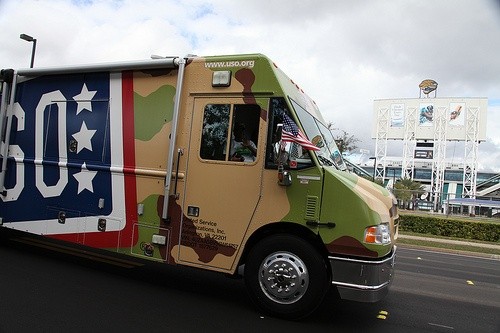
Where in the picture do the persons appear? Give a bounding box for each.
[224,119,258,162]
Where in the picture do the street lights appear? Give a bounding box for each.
[20,33,37,69]
[369,157,378,183]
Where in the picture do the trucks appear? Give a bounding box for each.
[0,53,400,322]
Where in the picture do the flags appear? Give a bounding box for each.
[282,112,325,151]
